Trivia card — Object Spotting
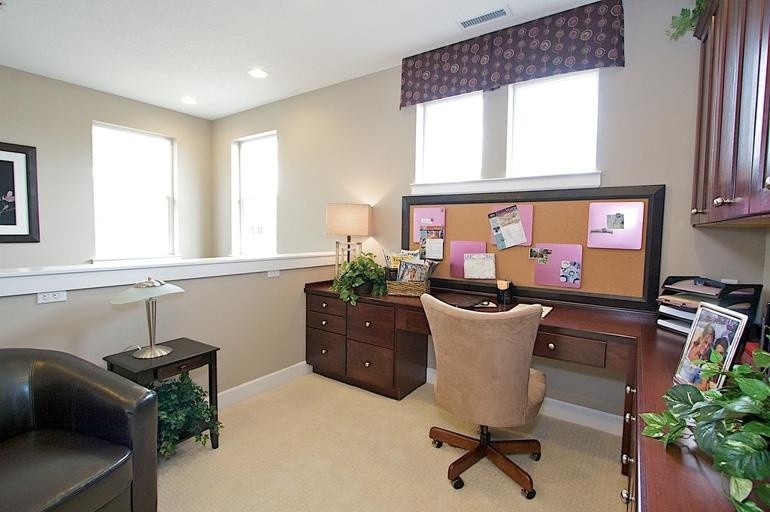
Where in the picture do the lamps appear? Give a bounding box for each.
[109,276,185,358]
[325,202,372,277]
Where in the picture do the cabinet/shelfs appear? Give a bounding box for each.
[690,0,770,229]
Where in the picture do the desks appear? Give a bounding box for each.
[302,273,770,512]
[102,336,221,451]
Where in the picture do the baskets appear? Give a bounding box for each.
[386,280,431,297]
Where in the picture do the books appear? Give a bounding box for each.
[657,279,752,333]
[385,249,441,282]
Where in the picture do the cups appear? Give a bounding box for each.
[497,280,512,305]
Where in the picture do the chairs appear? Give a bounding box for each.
[0,347,159,512]
[418,293,547,500]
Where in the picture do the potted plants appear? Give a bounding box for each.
[332,255,386,297]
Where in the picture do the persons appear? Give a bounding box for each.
[677,326,728,394]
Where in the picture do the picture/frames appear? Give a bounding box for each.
[671,301,747,395]
[0,140,42,244]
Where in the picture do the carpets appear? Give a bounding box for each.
[156,373,630,511]
